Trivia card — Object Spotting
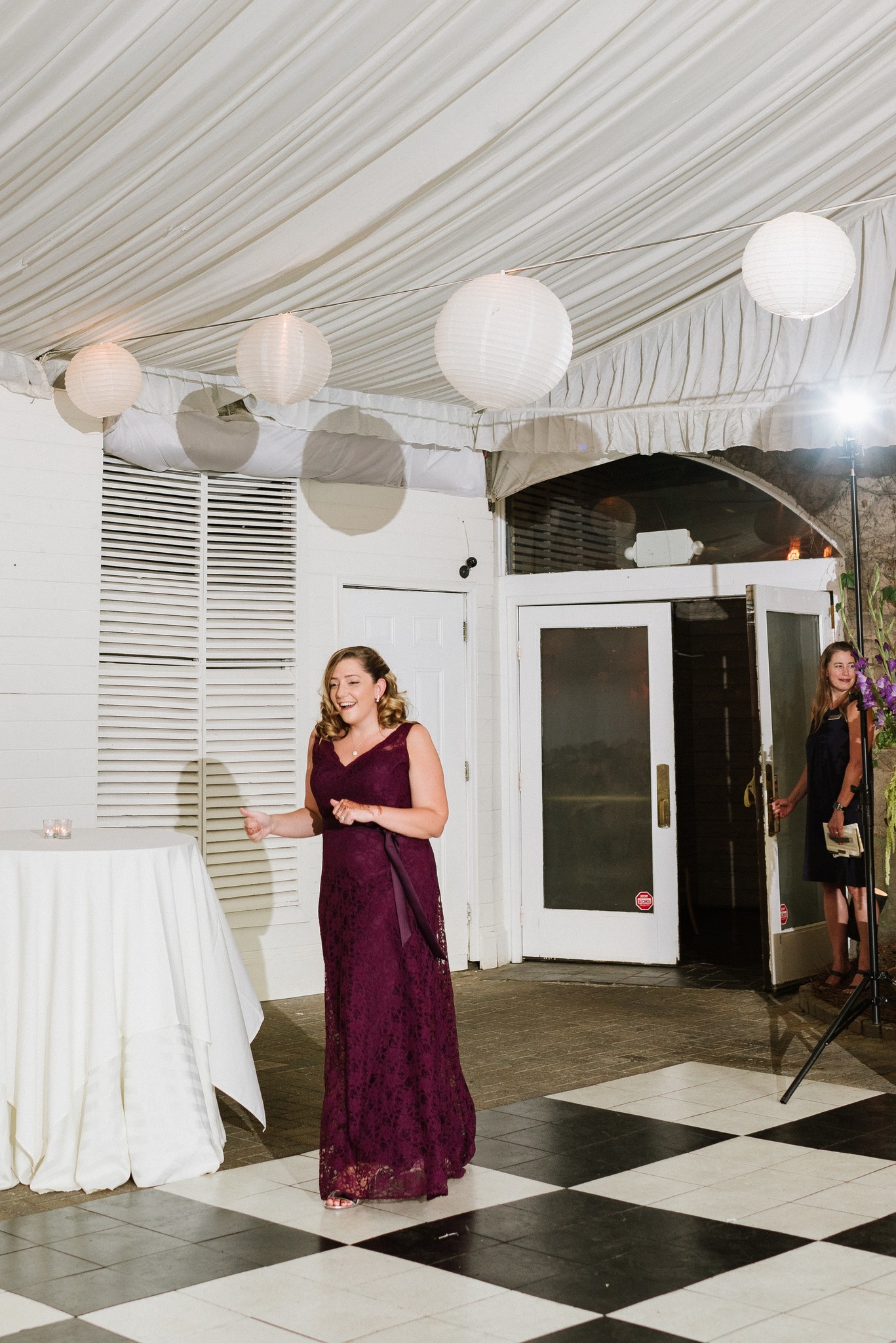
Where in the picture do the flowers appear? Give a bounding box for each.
[833,560,896,887]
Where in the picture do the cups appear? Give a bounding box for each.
[43,819,72,839]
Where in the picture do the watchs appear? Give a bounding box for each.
[832,801,848,812]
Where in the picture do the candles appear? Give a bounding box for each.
[42,818,73,840]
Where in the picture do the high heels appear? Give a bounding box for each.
[844,969,871,994]
[817,970,853,991]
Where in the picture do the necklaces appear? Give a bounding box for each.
[348,725,380,756]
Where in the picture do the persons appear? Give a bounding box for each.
[767,640,881,1002]
[235,643,480,1213]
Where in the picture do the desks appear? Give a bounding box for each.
[0,825,276,1201]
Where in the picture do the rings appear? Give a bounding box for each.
[339,815,345,819]
[834,831,838,834]
